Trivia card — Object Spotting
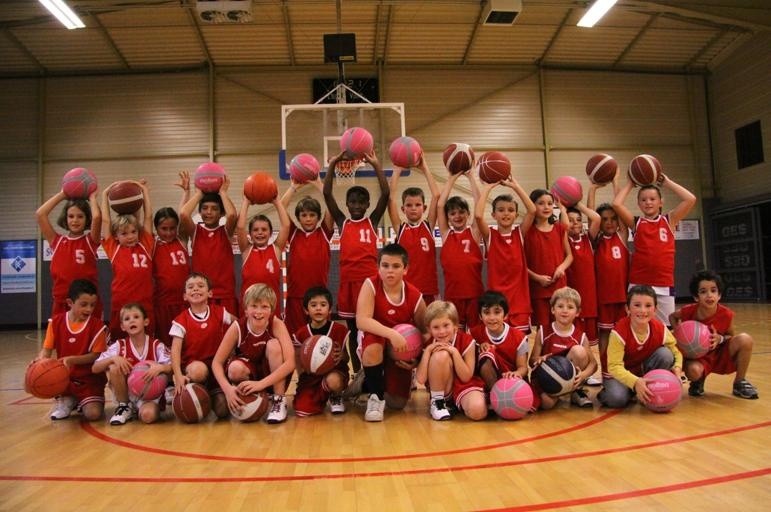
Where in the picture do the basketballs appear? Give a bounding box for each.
[627,153,662,188]
[585,153,618,185]
[673,320,713,360]
[243,173,278,206]
[490,376,534,420]
[339,127,374,160]
[386,323,423,364]
[108,181,144,215]
[300,335,338,377]
[231,389,269,423]
[194,162,227,193]
[62,167,98,200]
[537,355,578,397]
[641,369,683,413]
[477,151,512,184]
[442,142,475,176]
[388,137,423,169]
[171,382,211,425]
[25,358,70,399]
[126,359,168,401]
[290,152,320,184]
[550,175,583,208]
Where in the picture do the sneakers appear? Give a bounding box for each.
[429,397,451,421]
[267,394,289,423]
[733,380,757,398]
[331,396,346,414]
[365,394,386,421]
[346,369,365,397]
[587,376,602,384]
[51,395,74,420]
[165,384,176,403]
[110,402,135,424]
[690,383,703,397]
[571,389,594,408]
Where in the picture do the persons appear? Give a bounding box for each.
[474,173,537,332]
[211,283,295,422]
[92,304,172,425]
[290,286,348,418]
[25,278,107,421]
[466,289,541,415]
[100,180,154,341]
[528,287,598,408]
[594,285,683,407]
[179,176,237,317]
[416,300,488,421]
[35,186,102,318]
[388,148,440,305]
[343,243,426,421]
[323,149,390,331]
[168,271,237,418]
[554,198,601,344]
[139,170,191,349]
[281,171,334,340]
[668,269,758,398]
[437,160,485,328]
[613,173,696,329]
[525,188,574,326]
[587,166,629,346]
[237,184,290,321]
